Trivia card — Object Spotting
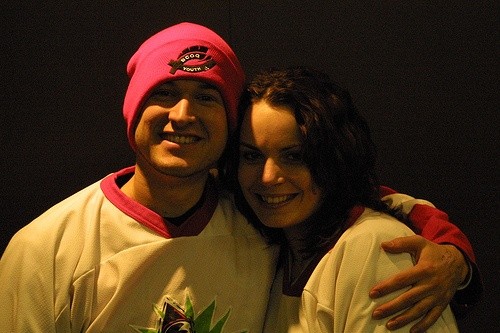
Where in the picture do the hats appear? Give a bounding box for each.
[124,22,244,155]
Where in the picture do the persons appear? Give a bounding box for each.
[217,65,461,333]
[0,21,484,333]
